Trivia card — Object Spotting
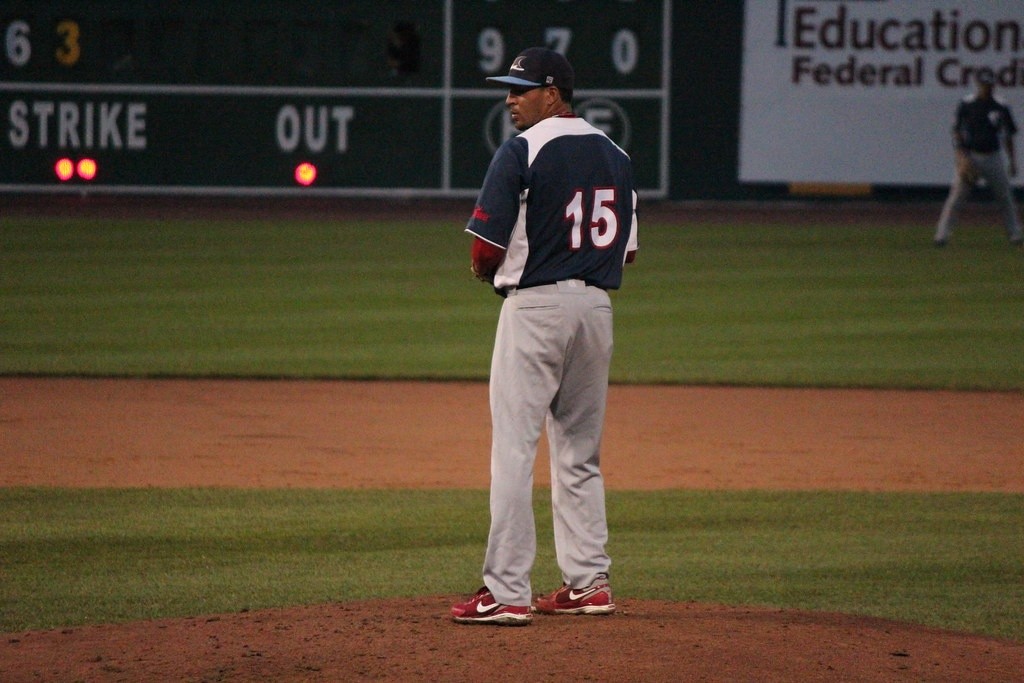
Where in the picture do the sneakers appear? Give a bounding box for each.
[535,574,616,615]
[449,586,534,626]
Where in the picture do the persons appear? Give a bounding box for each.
[449,49,640,625]
[930,67,1024,247]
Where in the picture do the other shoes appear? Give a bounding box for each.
[932,235,948,248]
[1010,235,1024,248]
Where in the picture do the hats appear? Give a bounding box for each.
[975,66,994,85]
[485,46,575,90]
